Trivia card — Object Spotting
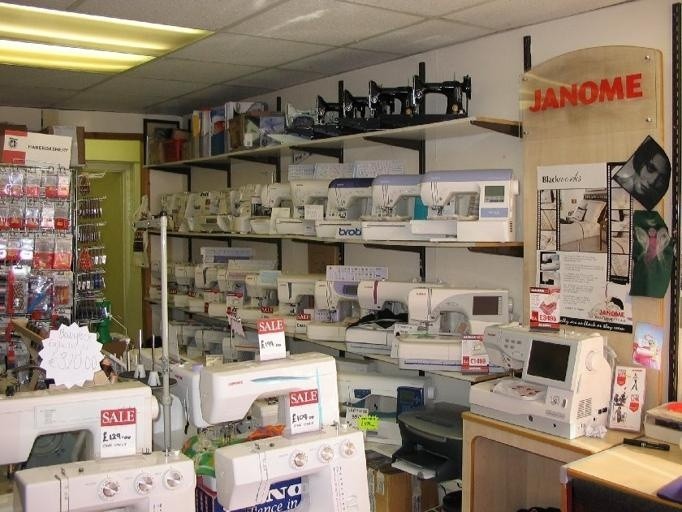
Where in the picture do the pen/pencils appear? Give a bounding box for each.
[623,438,669,450]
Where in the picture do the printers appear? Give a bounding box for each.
[390,403,469,483]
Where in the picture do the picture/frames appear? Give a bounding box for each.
[143,120,178,166]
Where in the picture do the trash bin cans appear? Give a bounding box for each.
[442,490,462,512]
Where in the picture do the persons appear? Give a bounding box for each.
[615,153,667,196]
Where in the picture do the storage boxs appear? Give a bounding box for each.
[367,457,440,510]
[2,123,85,170]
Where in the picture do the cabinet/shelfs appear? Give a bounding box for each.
[139,110,525,467]
[458,414,682,511]
